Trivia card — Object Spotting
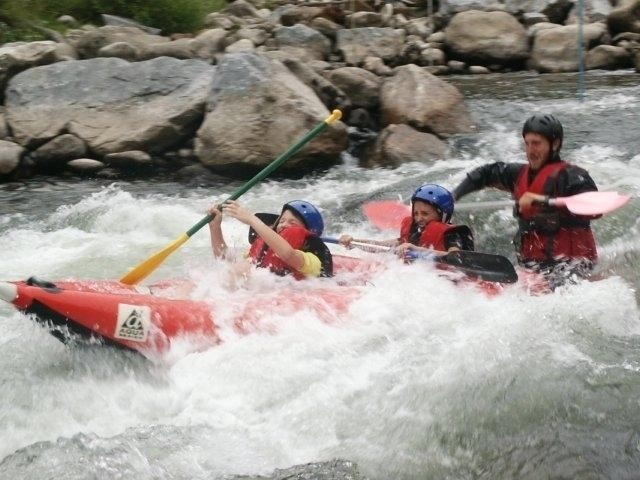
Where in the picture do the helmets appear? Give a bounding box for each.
[285,199,323,236]
[522,113,563,142]
[412,184,454,222]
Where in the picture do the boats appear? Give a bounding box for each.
[2,263,607,363]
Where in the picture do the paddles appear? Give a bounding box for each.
[120,108,342,285]
[248,213,517,283]
[364,191,634,230]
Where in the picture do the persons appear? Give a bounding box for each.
[206,200,323,291]
[339,185,466,273]
[451,112,602,280]
